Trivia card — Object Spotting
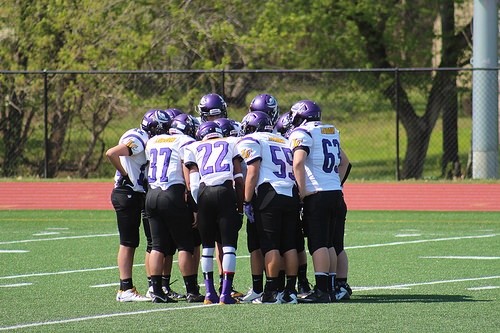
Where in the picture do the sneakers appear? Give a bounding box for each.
[116,289,355,304]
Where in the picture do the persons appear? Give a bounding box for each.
[106,110,172,302]
[237,111,300,305]
[145,114,205,303]
[184,121,244,305]
[288,99,343,304]
[142,94,352,301]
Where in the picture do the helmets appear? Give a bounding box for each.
[139,94,323,136]
